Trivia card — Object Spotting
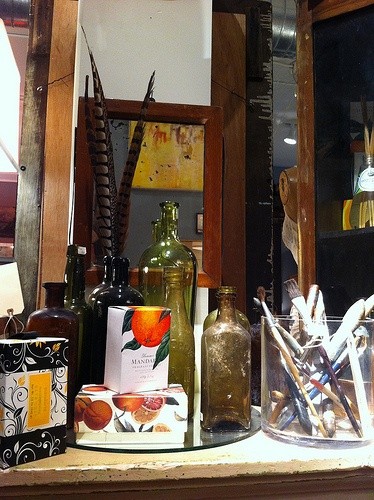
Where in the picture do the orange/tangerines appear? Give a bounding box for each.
[74,386,163,431]
[131,304,170,347]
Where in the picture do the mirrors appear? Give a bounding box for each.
[76,95,224,289]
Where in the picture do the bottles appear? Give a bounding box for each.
[201,292,252,432]
[87,255,115,309]
[138,201,198,329]
[94,257,145,382]
[202,286,250,332]
[348,154,374,228]
[63,244,94,392]
[162,265,195,420]
[25,282,79,429]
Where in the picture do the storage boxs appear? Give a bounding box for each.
[71,304,193,454]
[0,324,69,471]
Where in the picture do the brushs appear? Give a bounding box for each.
[256,286,330,439]
[268,284,374,438]
[284,278,364,439]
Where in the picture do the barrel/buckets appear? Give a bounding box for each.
[260,315,374,447]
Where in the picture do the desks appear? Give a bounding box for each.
[0,403,374,500]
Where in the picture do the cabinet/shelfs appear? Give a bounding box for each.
[291,0,374,314]
